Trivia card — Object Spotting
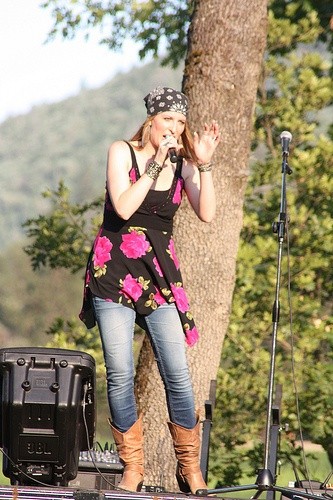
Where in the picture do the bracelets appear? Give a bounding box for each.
[195,161,214,172]
[145,159,162,181]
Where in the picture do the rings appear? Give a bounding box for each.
[211,138,217,141]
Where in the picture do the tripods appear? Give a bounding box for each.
[196,152,331,500]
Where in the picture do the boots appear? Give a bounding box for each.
[107,411,143,492]
[167,415,208,496]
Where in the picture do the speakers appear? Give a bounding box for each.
[0,347,96,487]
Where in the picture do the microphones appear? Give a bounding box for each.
[165,135,178,163]
[279,130,293,172]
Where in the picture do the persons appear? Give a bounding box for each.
[77,87,224,497]
